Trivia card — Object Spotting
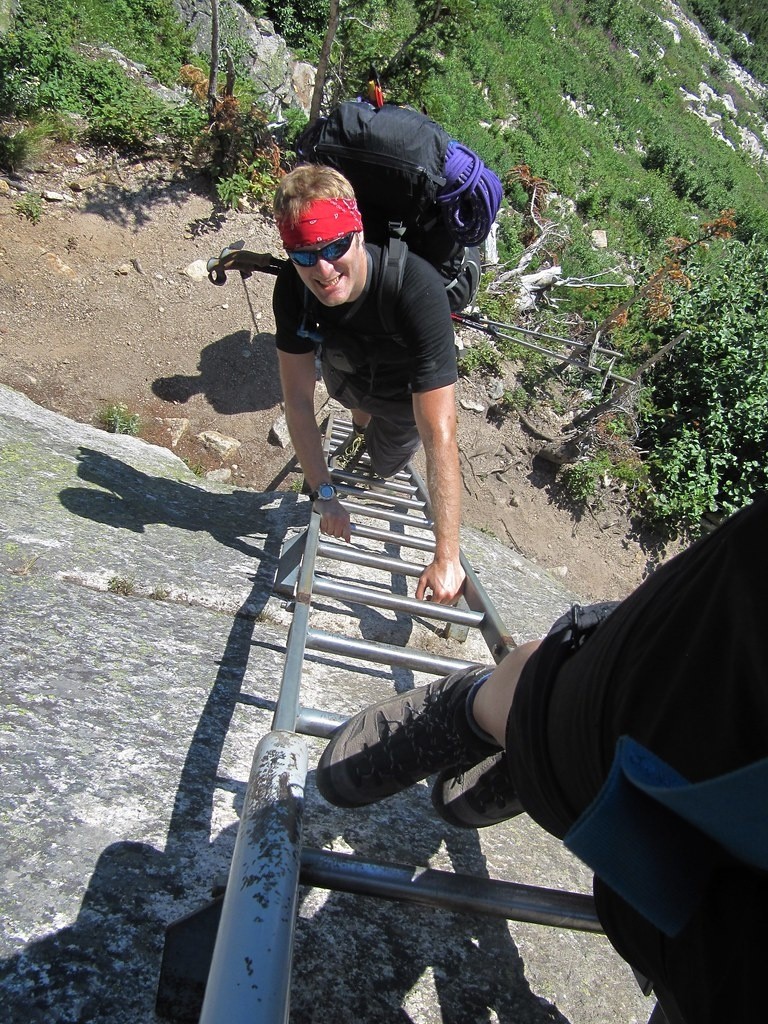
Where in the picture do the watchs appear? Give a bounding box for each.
[309,482,337,501]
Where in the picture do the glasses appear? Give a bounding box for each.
[283,232,355,267]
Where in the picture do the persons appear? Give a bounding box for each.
[313,496,768,1024]
[265,163,467,606]
[294,91,482,312]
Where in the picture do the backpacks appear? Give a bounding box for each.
[296,101,503,313]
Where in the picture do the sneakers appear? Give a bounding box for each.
[369,470,389,494]
[431,747,526,829]
[316,664,507,808]
[329,431,368,474]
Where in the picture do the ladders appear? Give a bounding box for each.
[193,408,671,1024]
